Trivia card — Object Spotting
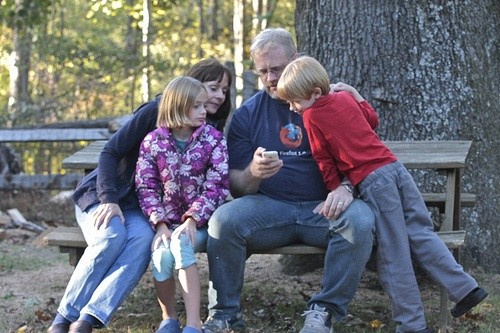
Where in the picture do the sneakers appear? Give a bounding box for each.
[299,302,334,333]
[200,310,242,333]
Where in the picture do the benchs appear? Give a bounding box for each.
[44,192,478,254]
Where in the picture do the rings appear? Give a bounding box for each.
[338,202,344,205]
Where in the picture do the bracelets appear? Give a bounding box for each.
[339,183,358,198]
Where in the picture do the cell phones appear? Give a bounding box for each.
[262,151,279,161]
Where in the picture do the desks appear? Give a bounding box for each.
[62,140,472,333]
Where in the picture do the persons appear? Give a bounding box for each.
[132,75,230,333]
[200,27,376,333]
[43,58,233,333]
[276,56,490,333]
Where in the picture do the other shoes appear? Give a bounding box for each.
[182,325,201,333]
[47,323,68,333]
[156,320,181,333]
[450,286,489,318]
[410,328,429,333]
[255,55,291,76]
[68,320,92,333]
